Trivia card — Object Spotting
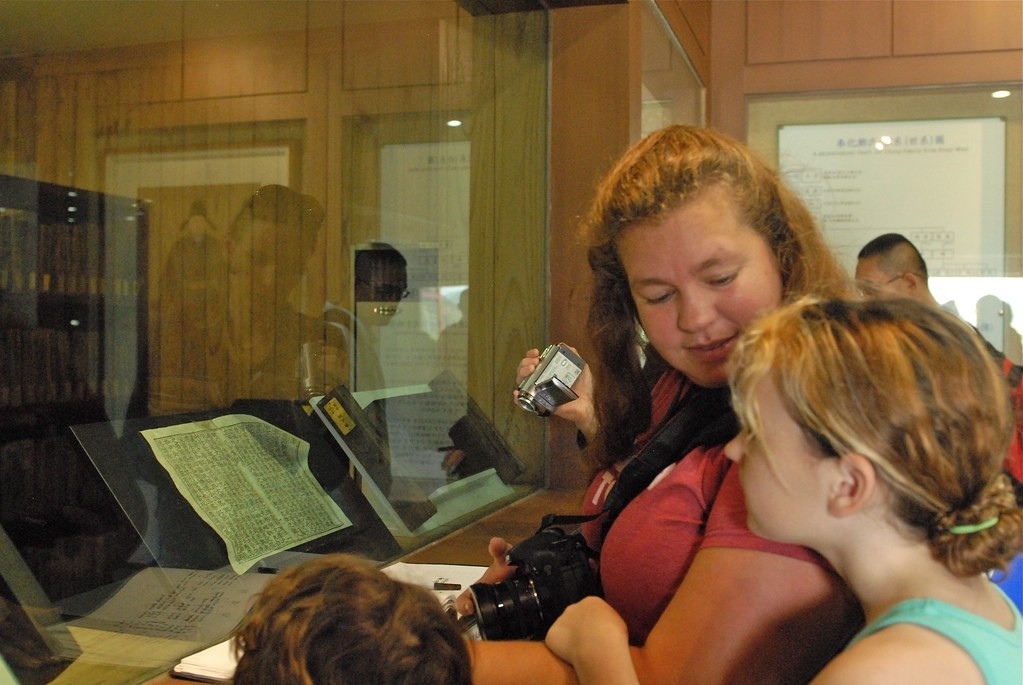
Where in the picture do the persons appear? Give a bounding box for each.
[232,558,474,685]
[546,289,1023,685]
[856,233,986,345]
[117,184,392,574]
[454,124,864,685]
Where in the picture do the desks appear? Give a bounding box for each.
[148,489,588,685]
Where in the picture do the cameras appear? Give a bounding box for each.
[468,527,596,644]
[516,340,586,419]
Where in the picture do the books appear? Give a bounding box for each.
[169,560,490,685]
